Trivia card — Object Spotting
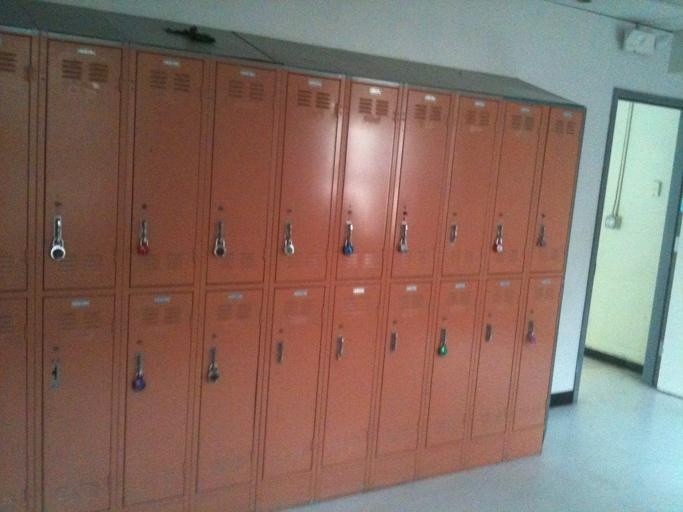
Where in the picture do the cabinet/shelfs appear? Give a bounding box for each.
[0,0,587,512]
